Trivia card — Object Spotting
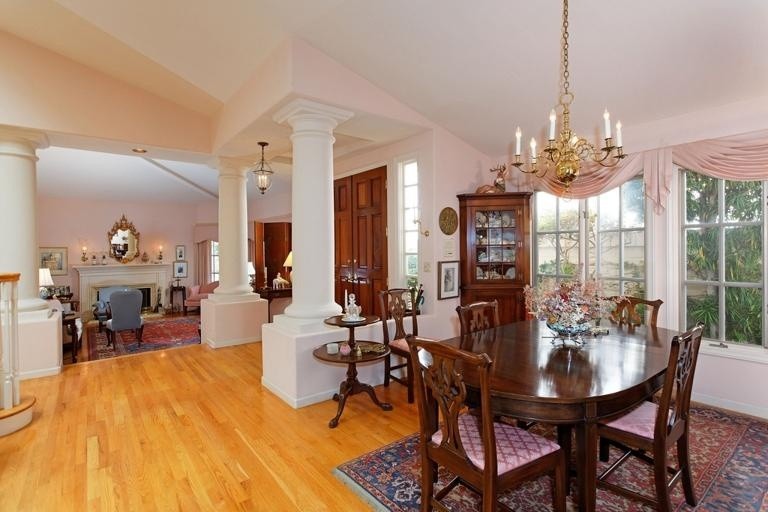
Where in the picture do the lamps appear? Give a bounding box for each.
[253,141,274,195]
[513,0,629,193]
[283,250,293,283]
[37,268,54,297]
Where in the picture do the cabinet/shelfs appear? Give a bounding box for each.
[460,190,532,323]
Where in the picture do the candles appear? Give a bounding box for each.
[83,247,87,253]
[159,246,162,252]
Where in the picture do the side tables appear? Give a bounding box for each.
[63,314,83,363]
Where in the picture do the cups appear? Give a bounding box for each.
[54,289,60,296]
[325,343,340,354]
[64,288,70,295]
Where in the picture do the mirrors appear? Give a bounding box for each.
[108,216,140,264]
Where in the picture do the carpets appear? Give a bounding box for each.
[331,396,768,512]
[86,318,201,360]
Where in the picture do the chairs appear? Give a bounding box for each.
[92,286,144,350]
[593,294,664,405]
[454,299,540,431]
[403,334,568,511]
[594,321,705,511]
[183,281,219,314]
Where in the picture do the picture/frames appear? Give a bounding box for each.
[38,246,69,276]
[438,261,460,300]
[173,245,189,278]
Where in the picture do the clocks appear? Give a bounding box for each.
[439,206,458,235]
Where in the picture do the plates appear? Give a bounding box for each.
[476,267,515,280]
[475,212,518,263]
[341,317,366,322]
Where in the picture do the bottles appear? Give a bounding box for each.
[340,342,351,356]
[91,256,96,266]
[102,255,107,265]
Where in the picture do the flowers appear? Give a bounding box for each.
[522,280,617,334]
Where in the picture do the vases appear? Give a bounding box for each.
[545,321,587,347]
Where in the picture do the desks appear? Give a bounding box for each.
[416,317,686,511]
[313,314,392,428]
